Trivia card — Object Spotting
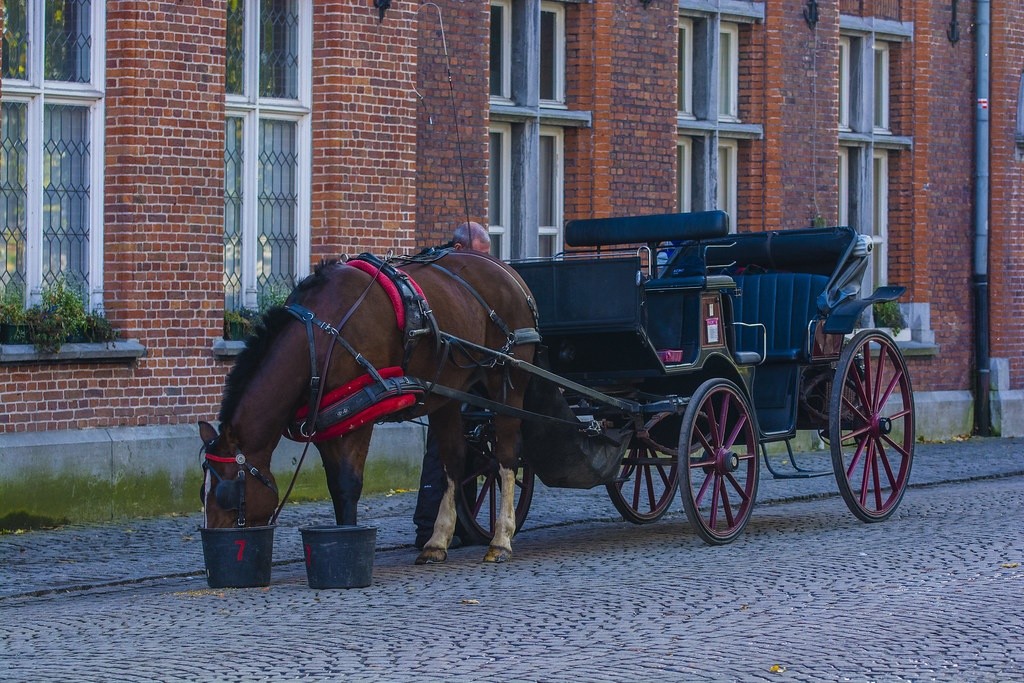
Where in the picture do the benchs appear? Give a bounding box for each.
[729,273,829,362]
[722,291,766,366]
[564,210,735,296]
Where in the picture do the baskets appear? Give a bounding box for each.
[797,375,854,426]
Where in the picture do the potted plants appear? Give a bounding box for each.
[65,292,92,343]
[224,311,249,341]
[0,293,30,344]
[872,282,909,337]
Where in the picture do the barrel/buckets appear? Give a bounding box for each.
[198,524,278,588]
[299,525,378,589]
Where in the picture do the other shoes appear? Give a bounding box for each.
[463,535,482,545]
[416,535,461,548]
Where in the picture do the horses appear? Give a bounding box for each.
[199,247,537,564]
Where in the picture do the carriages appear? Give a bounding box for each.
[196,210,917,566]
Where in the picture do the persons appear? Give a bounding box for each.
[411,219,495,548]
